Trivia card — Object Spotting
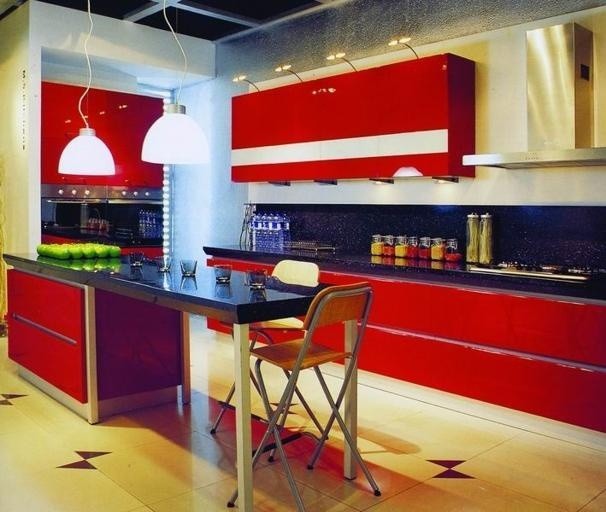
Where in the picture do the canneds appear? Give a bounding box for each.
[371,235,462,263]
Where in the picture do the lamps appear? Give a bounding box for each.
[58,0,115,175]
[139,1,210,164]
[231,37,420,92]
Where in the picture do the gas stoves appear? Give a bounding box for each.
[469,256,598,285]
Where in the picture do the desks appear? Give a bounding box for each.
[2,243,359,510]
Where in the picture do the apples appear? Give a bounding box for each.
[37,243,121,259]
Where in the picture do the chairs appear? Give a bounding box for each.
[227,281,379,512]
[208,260,329,462]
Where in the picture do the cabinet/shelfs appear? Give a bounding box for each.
[231,52,477,186]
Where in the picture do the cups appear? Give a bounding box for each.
[127,267,143,281]
[214,284,233,300]
[248,288,268,305]
[180,277,198,291]
[152,254,172,272]
[128,251,144,266]
[178,259,197,276]
[154,271,171,285]
[247,267,266,288]
[212,264,231,284]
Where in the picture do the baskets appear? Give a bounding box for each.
[285,239,335,250]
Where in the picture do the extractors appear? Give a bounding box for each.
[460,22,606,172]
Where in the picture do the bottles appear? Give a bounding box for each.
[370,213,498,272]
[136,210,163,244]
[247,211,294,255]
[83,217,113,236]
